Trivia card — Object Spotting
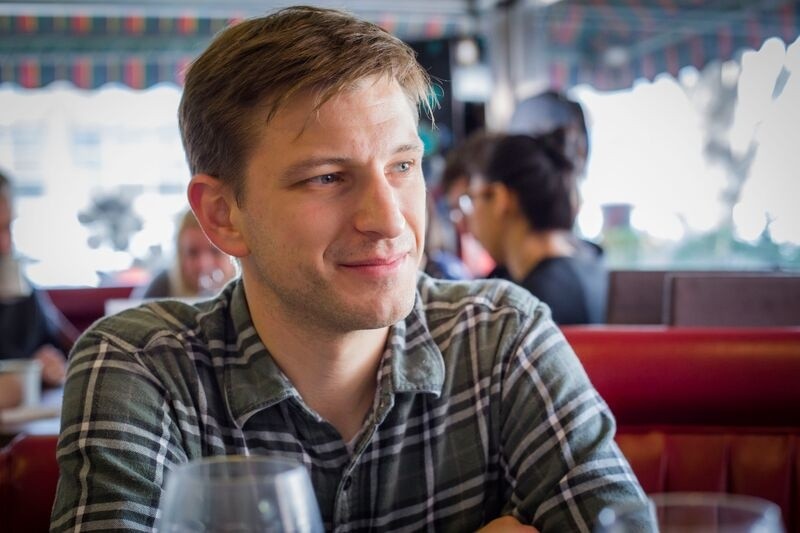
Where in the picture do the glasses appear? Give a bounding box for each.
[458,184,493,215]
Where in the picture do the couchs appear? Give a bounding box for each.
[558,322,800,532]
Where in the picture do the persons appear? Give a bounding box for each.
[136,206,238,301]
[49,5,658,532]
[0,172,71,415]
[459,126,610,326]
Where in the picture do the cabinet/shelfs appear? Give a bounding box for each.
[609,271,799,327]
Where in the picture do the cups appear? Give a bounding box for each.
[151,457,324,533]
[595,492,783,533]
[2,357,44,408]
